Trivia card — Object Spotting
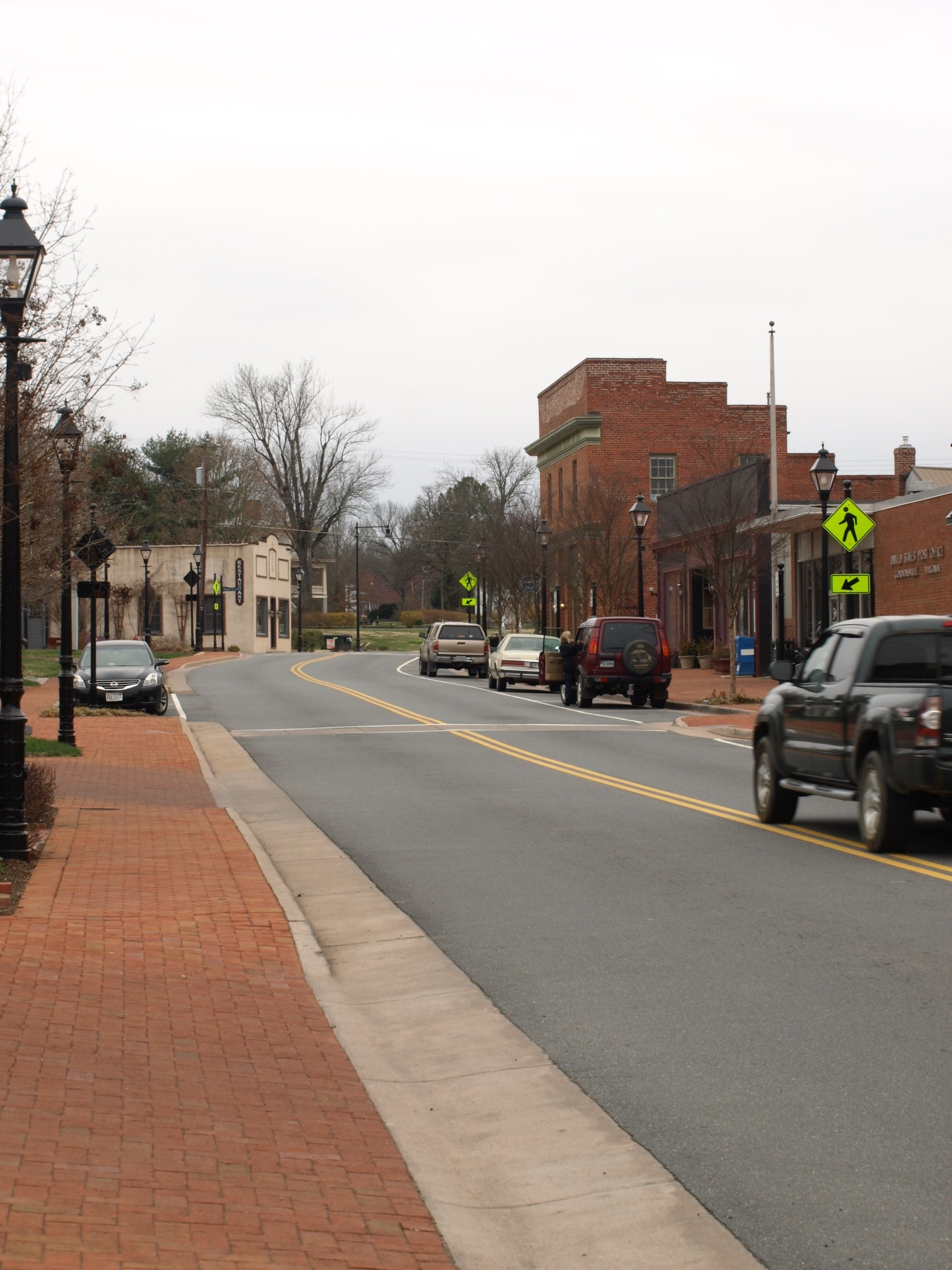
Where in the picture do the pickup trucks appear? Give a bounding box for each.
[752,613,952,854]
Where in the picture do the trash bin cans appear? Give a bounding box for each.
[735,637,754,675]
[489,636,504,653]
[324,635,352,651]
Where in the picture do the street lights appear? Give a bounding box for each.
[473,550,482,624]
[808,441,839,630]
[354,522,392,652]
[192,543,203,652]
[140,537,152,648]
[627,491,653,618]
[536,515,554,635]
[295,563,305,652]
[0,172,48,863]
[47,395,87,751]
[477,534,488,637]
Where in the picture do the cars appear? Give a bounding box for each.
[487,633,565,693]
[72,640,170,716]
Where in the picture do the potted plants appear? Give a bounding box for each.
[678,640,697,669]
[695,635,714,669]
[712,640,732,672]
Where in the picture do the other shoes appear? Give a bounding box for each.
[565,702,570,706]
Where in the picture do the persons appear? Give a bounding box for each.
[559,631,580,707]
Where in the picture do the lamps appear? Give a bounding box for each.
[677,581,682,594]
[649,587,657,596]
[669,587,673,596]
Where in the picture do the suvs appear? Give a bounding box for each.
[538,615,672,709]
[419,618,491,679]
